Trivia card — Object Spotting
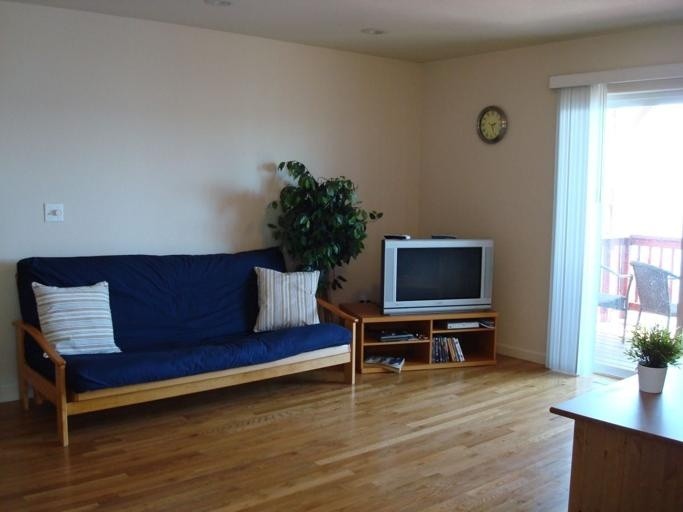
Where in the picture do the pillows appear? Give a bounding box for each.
[29,279,122,359]
[252,265,323,334]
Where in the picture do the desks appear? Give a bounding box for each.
[549,367,683,512]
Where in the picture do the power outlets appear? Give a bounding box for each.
[43,204,66,225]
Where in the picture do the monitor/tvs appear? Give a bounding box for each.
[384,239,494,315]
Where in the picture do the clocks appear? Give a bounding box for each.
[477,106,508,147]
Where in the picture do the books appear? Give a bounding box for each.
[432,337,464,363]
[447,319,479,329]
[380,355,405,373]
[365,354,383,363]
[479,320,494,328]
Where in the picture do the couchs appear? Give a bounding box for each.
[12,245,359,446]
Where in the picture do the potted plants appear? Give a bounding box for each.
[629,329,683,394]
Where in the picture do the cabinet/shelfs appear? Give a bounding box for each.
[337,303,498,375]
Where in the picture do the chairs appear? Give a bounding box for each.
[599,265,634,344]
[630,261,680,334]
